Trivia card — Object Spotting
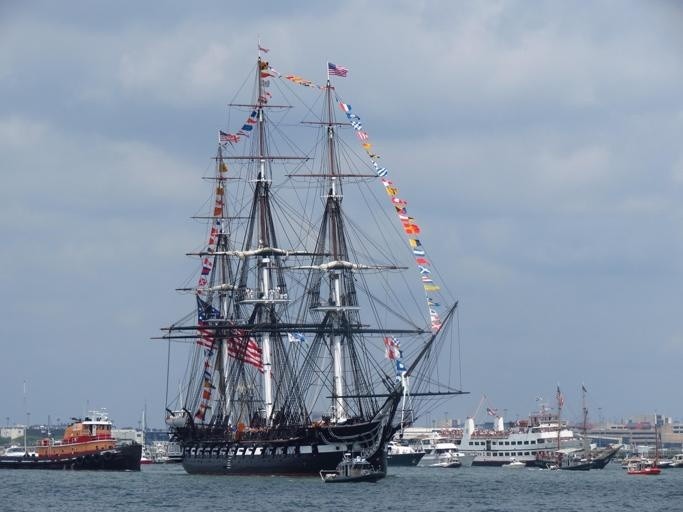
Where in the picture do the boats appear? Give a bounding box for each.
[3,443,40,457]
[112,428,184,467]
[0,403,146,471]
[384,378,683,475]
[319,442,389,483]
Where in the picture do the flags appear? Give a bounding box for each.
[289,332,305,342]
[487,407,496,416]
[329,62,441,330]
[383,336,409,389]
[195,159,264,419]
[217,47,326,143]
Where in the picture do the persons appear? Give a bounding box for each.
[224,423,266,442]
[438,418,668,472]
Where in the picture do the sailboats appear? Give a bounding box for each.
[146,34,473,483]
